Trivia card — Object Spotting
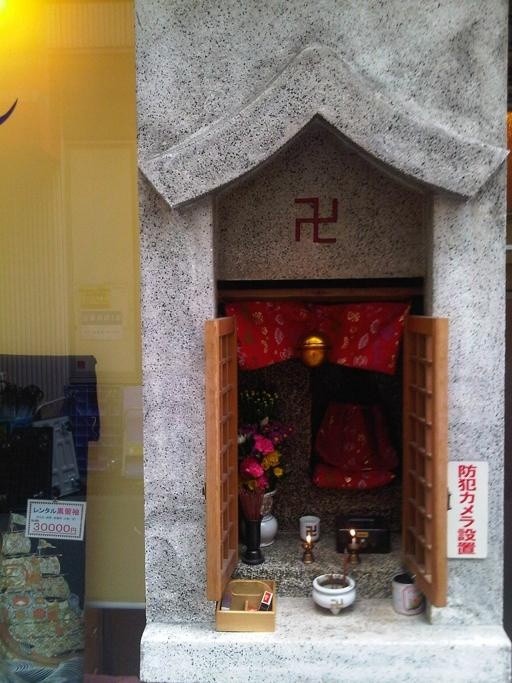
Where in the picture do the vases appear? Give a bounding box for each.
[240,515,265,565]
[260,489,278,547]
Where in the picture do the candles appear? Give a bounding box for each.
[306,535,311,545]
[350,528,357,544]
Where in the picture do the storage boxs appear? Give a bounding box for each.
[336,515,390,553]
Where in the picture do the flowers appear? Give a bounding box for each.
[238,423,296,521]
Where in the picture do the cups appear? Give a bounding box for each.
[391,574,425,615]
[300,515,320,542]
